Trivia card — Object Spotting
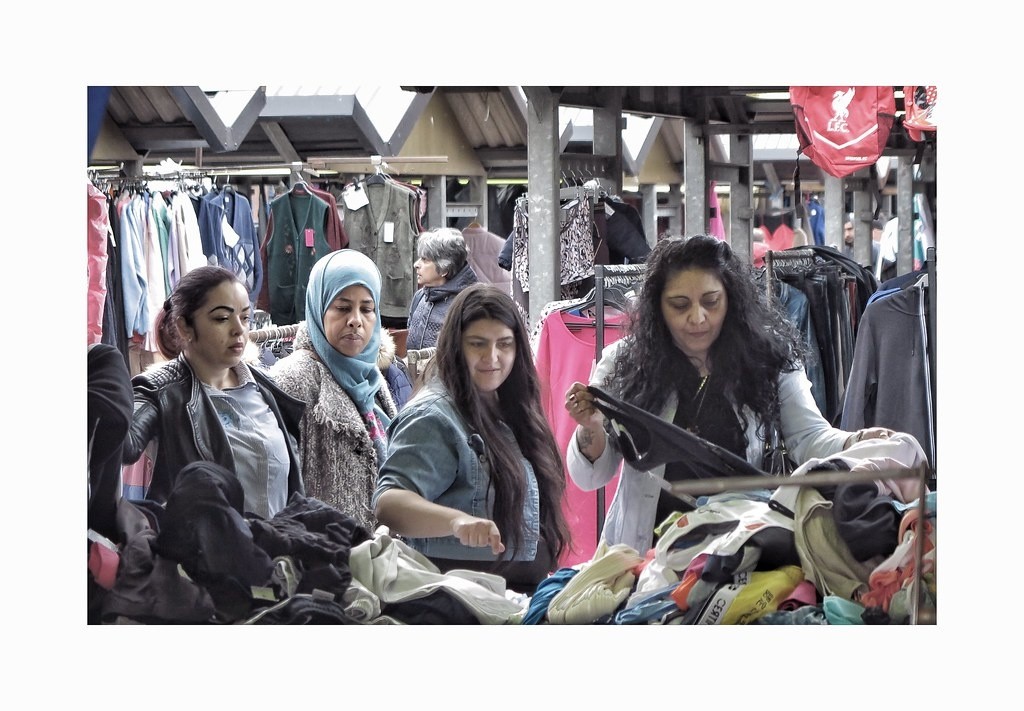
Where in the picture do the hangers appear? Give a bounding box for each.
[516,166,628,216]
[253,321,300,363]
[92,168,422,208]
[750,249,861,305]
[546,264,649,333]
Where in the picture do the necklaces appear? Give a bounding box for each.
[674,363,716,438]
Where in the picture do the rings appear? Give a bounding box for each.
[570,394,577,404]
[575,403,582,413]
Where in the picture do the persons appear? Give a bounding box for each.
[88,342,137,553]
[261,249,400,545]
[750,229,769,269]
[402,228,481,363]
[563,233,901,564]
[839,213,881,272]
[116,266,307,523]
[375,280,569,601]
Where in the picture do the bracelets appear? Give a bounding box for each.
[856,428,867,442]
